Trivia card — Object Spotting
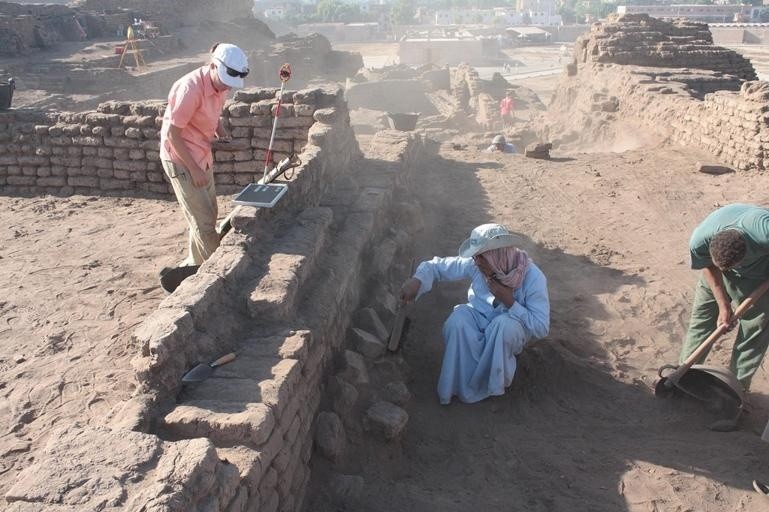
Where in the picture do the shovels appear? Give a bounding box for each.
[181,352,236,382]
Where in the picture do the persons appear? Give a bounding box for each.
[396,222,552,406]
[485,133,520,154]
[659,199,769,417]
[158,41,251,268]
[499,90,516,131]
[502,61,520,76]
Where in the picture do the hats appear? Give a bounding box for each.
[211,43,248,88]
[505,92,510,95]
[459,223,521,258]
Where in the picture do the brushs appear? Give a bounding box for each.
[386,258,415,353]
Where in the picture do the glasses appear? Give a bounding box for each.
[214,57,249,79]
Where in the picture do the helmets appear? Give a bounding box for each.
[492,135,506,144]
